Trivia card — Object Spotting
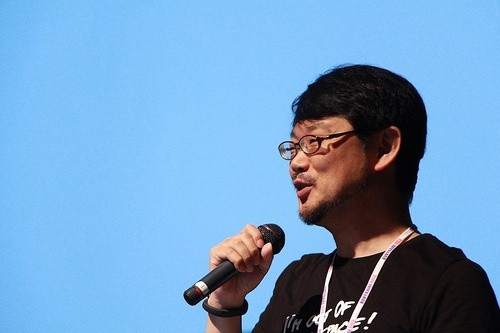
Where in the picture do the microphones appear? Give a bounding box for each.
[182,223,285,306]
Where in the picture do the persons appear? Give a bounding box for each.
[203,64,499,333]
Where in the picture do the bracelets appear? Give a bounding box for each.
[199,298,249,319]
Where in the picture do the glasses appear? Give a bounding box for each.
[279,123,389,160]
